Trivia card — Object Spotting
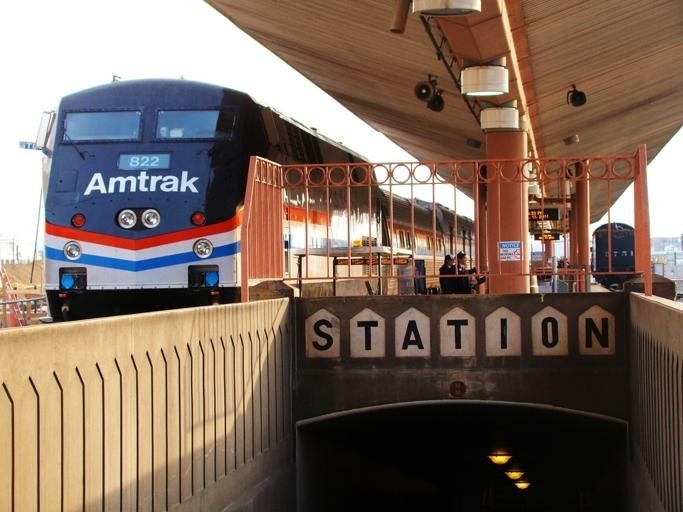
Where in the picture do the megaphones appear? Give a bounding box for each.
[414,79,437,101]
[427,89,444,112]
[570,90,586,107]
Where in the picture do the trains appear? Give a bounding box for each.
[44,79,476,324]
[591,222,635,289]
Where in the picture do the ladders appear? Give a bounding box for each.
[0,268,25,327]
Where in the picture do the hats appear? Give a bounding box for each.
[446,255,454,260]
[458,251,466,257]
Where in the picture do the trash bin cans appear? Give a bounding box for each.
[568,264,588,293]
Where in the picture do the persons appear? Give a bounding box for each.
[445,250,485,294]
[438,254,455,294]
[557,258,569,269]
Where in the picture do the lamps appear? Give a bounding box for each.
[460,56,509,96]
[480,100,519,130]
[412,0,481,16]
[488,454,531,491]
